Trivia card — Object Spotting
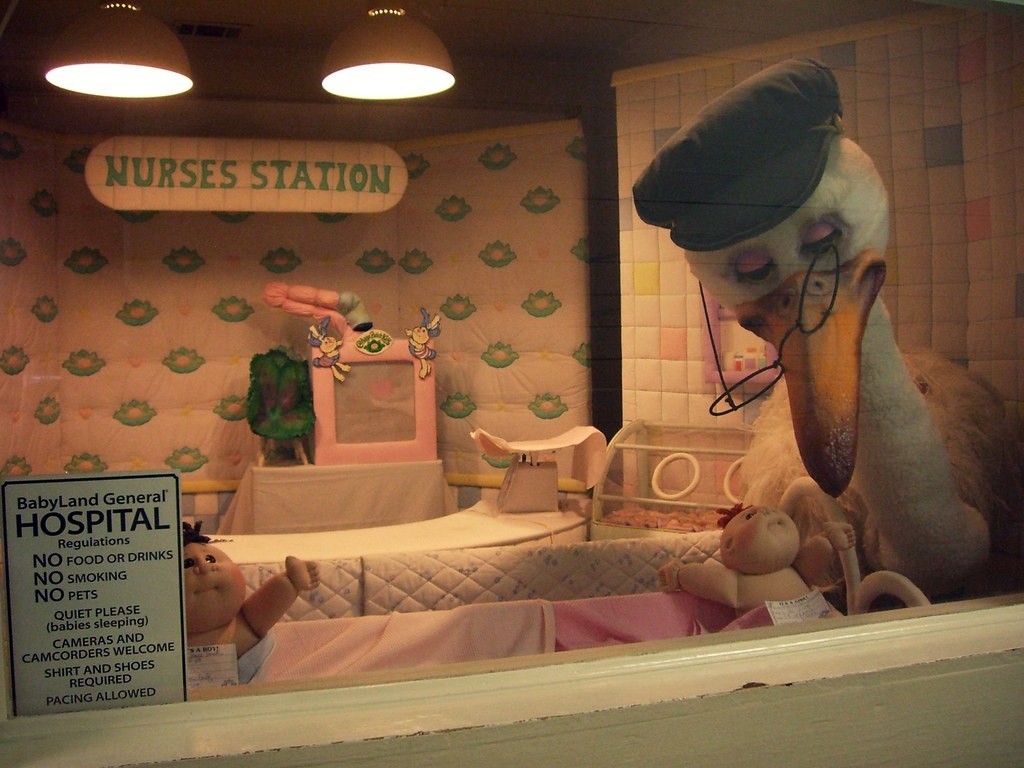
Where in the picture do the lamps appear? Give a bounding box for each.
[321,4,456,101]
[44,1,193,99]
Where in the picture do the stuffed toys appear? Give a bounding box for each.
[633,58,1015,621]
[181,516,321,658]
